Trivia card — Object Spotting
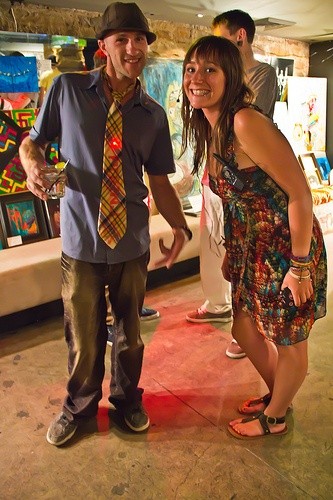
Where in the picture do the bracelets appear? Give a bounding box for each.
[288,253,311,285]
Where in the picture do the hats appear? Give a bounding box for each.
[96,2,156,45]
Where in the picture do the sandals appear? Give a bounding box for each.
[237,393,293,415]
[227,411,288,439]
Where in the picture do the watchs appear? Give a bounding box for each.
[178,225,192,242]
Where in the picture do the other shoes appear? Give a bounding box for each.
[123,403,150,431]
[106,325,114,346]
[139,307,159,320]
[46,411,80,445]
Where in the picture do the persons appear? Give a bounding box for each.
[184,9,277,359]
[177,36,326,438]
[18,1,193,446]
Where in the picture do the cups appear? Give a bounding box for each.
[42,173,65,199]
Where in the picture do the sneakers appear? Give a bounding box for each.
[226,339,247,358]
[185,305,232,323]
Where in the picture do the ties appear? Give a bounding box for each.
[97,67,137,249]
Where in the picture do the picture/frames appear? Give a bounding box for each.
[298,153,325,190]
[43,198,60,238]
[0,191,49,248]
